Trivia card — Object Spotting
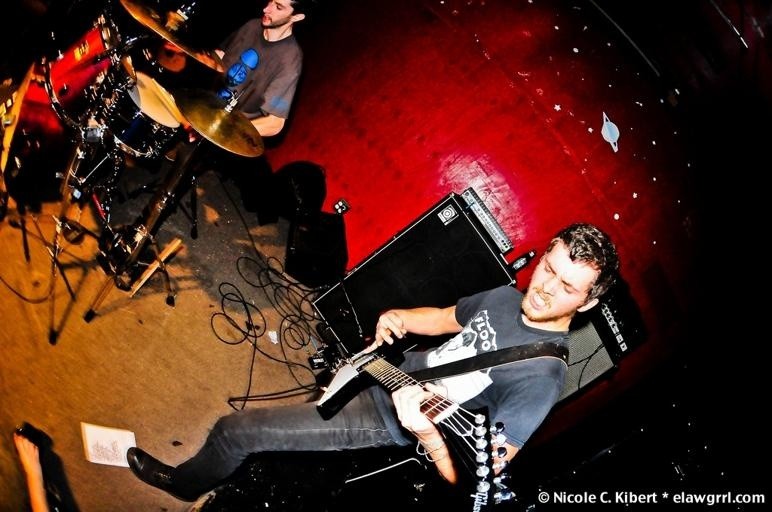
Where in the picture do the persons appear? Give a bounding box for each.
[164,0,308,162]
[127,225,621,503]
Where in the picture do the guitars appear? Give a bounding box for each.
[315,328,523,512]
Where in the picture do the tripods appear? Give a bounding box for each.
[70,136,203,323]
[0,32,157,345]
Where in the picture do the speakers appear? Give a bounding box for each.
[310,191,517,376]
[554,311,619,411]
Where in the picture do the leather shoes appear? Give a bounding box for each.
[127,447,198,502]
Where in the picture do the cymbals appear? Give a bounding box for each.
[121,0,228,75]
[174,84,265,158]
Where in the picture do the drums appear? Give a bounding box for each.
[41,9,136,129]
[130,40,188,83]
[91,72,183,164]
[0,58,76,204]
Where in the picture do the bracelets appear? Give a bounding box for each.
[415,439,448,462]
